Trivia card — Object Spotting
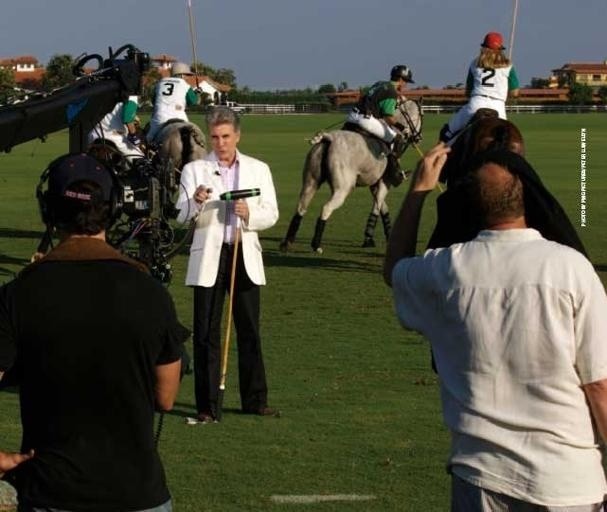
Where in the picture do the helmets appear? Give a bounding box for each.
[483,33,502,50]
[390,66,414,83]
[171,62,195,77]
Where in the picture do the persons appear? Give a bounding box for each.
[174,107,284,425]
[87,93,146,176]
[438,32,519,145]
[341,66,414,189]
[380,139,607,511]
[0,153,181,511]
[146,62,200,151]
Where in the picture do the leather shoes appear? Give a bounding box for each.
[199,413,211,423]
[254,407,272,414]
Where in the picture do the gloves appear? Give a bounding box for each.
[127,133,140,145]
[401,127,408,140]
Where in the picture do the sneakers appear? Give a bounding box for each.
[392,171,411,187]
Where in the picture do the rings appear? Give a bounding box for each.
[196,190,200,197]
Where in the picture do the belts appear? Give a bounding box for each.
[352,108,363,115]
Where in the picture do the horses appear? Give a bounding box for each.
[279,100,423,250]
[440,108,526,192]
[145,119,207,168]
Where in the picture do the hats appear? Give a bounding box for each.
[48,153,112,201]
